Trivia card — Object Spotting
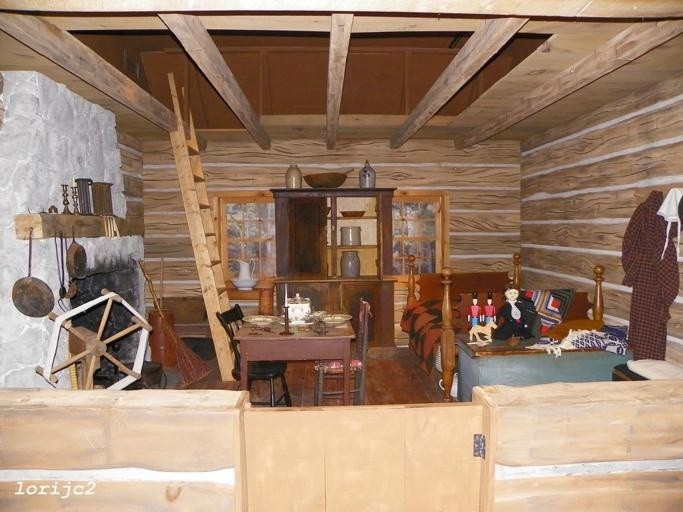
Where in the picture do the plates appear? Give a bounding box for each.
[241,315,281,326]
[278,319,315,327]
[321,313,352,324]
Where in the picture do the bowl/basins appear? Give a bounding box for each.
[230,279,259,291]
[302,171,347,188]
[340,210,365,218]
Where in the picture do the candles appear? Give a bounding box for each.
[70,175,77,188]
[282,282,288,307]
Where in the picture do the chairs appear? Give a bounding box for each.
[213,302,293,408]
[312,295,372,406]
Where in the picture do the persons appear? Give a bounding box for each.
[465,290,482,328]
[482,290,496,330]
[493,283,534,340]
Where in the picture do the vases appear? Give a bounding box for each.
[149,308,180,368]
[284,164,303,189]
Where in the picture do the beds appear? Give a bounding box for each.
[396,248,607,402]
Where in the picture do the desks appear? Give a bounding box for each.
[224,276,277,317]
[452,334,634,403]
[230,315,356,407]
[610,363,648,385]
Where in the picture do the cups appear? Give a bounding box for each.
[340,226,361,246]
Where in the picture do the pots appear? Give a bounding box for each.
[66,225,88,280]
[13,228,57,319]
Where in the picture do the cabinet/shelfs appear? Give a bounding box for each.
[276,281,392,354]
[269,186,398,281]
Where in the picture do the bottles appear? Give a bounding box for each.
[286,164,302,188]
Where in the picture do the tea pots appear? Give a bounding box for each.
[236,258,256,280]
